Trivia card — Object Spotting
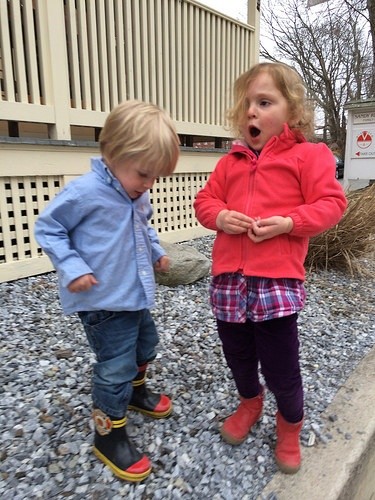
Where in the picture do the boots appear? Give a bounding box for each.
[127,365,172,419]
[221,385,264,445]
[92,407,152,482]
[274,410,304,474]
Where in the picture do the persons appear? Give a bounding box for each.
[36,101,174,482]
[193,63,346,475]
